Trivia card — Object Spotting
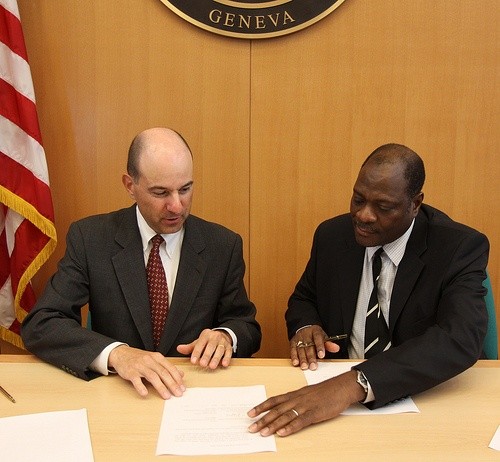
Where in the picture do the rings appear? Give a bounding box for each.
[297,341,302,346]
[292,410,298,417]
[219,345,224,347]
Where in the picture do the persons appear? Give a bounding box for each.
[246,143,490,436]
[21,127,262,400]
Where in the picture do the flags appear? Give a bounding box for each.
[0,0,58,350]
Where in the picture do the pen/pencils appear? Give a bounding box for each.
[299,334,347,348]
[0,386,16,403]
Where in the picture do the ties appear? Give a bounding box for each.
[145,234,169,352]
[363,247,389,359]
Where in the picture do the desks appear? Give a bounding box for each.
[0,355,500,462]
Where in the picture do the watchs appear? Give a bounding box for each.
[357,370,368,392]
